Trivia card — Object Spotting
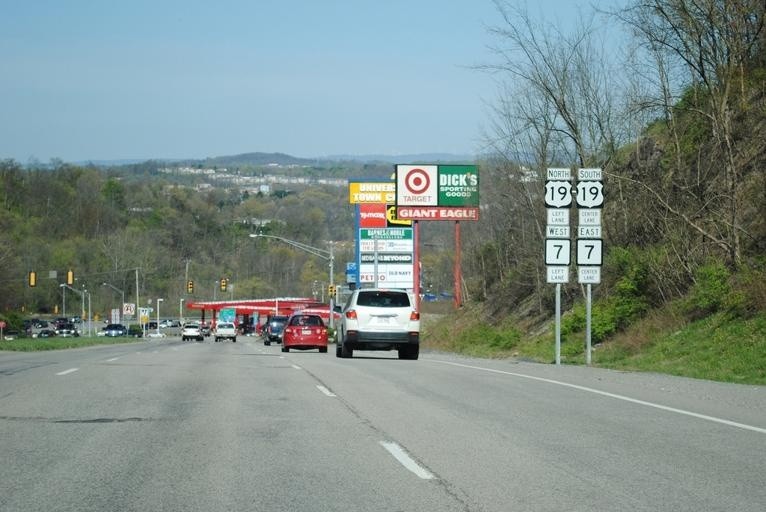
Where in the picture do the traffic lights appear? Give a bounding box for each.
[187,280,193,294]
[328,285,336,297]
[220,279,227,292]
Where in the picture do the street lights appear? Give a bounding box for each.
[60,284,85,320]
[103,282,125,326]
[249,231,339,328]
[156,299,164,334]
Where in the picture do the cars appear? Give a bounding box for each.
[181,324,210,341]
[336,288,420,359]
[96,324,143,337]
[262,314,328,352]
[214,322,238,342]
[23,316,81,338]
